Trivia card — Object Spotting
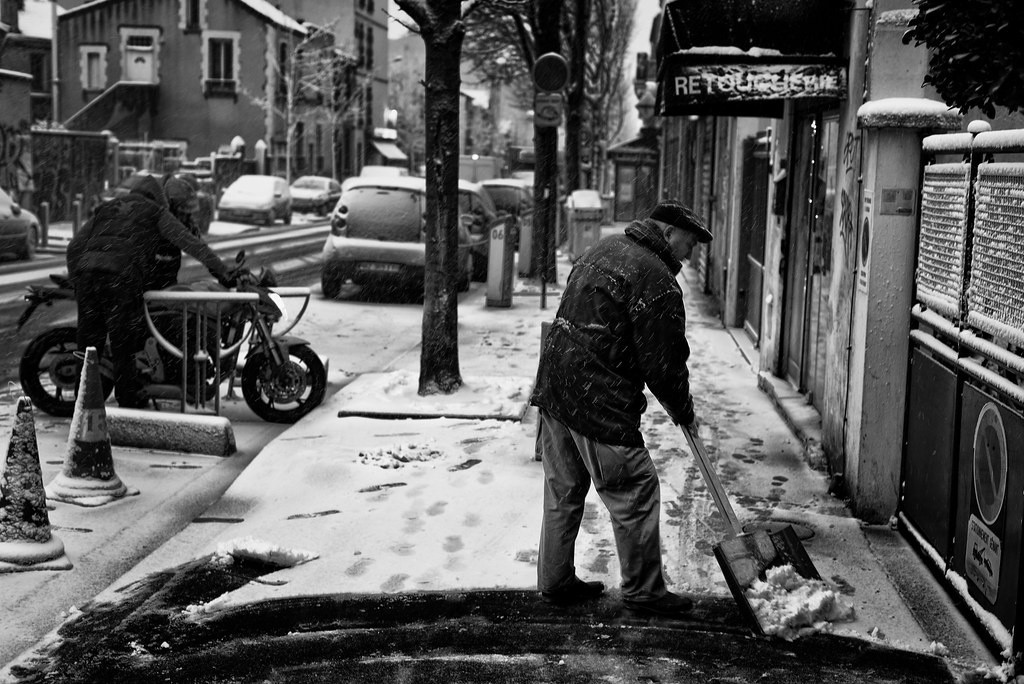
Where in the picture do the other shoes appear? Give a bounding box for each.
[119,404,154,410]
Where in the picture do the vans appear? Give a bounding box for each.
[458,153,502,184]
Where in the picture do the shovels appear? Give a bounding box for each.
[681,423,824,638]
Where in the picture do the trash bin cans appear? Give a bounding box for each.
[563,189,604,261]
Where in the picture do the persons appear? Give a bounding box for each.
[66,174,241,408]
[530,199,712,614]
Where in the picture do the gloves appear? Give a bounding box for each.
[219,267,249,288]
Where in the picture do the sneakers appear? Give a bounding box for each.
[621,591,696,619]
[541,577,604,603]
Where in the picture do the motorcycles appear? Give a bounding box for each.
[16,249,328,424]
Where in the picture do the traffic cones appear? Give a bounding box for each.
[0,396,74,576]
[44,346,141,507]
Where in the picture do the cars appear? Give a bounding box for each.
[322,173,476,304]
[454,177,536,283]
[0,186,42,261]
[217,173,293,227]
[291,175,342,216]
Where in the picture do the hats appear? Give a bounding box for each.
[650,200,714,244]
[132,176,170,208]
